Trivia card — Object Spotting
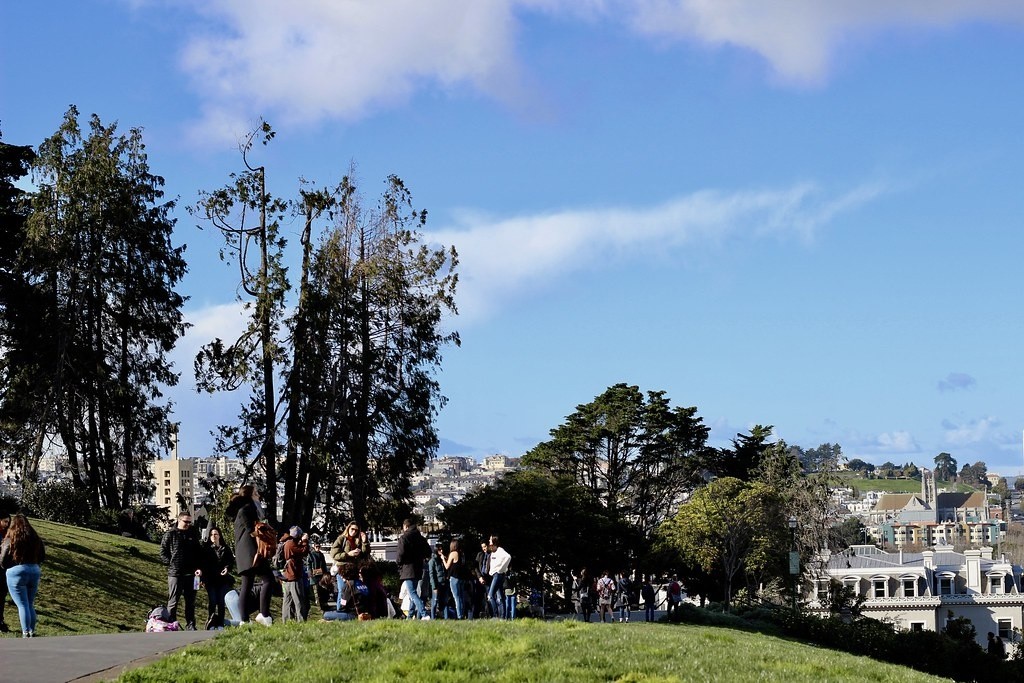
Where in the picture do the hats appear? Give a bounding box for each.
[289,526,303,537]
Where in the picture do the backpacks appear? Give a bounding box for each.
[600,578,612,600]
[272,538,293,571]
[148,604,170,621]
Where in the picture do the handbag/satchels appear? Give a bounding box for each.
[671,592,682,602]
[620,593,636,605]
[253,523,276,559]
[330,558,339,576]
[312,567,323,576]
[146,618,180,633]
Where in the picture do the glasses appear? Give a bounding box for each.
[438,548,443,551]
[180,520,192,524]
[316,546,321,547]
[352,528,359,532]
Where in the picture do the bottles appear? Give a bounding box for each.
[193,572,200,590]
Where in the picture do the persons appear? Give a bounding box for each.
[118,508,150,541]
[0,513,45,637]
[159,485,682,629]
[396,519,432,620]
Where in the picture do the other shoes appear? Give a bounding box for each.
[619,617,630,622]
[23,631,35,638]
[611,616,616,623]
[206,613,216,630]
